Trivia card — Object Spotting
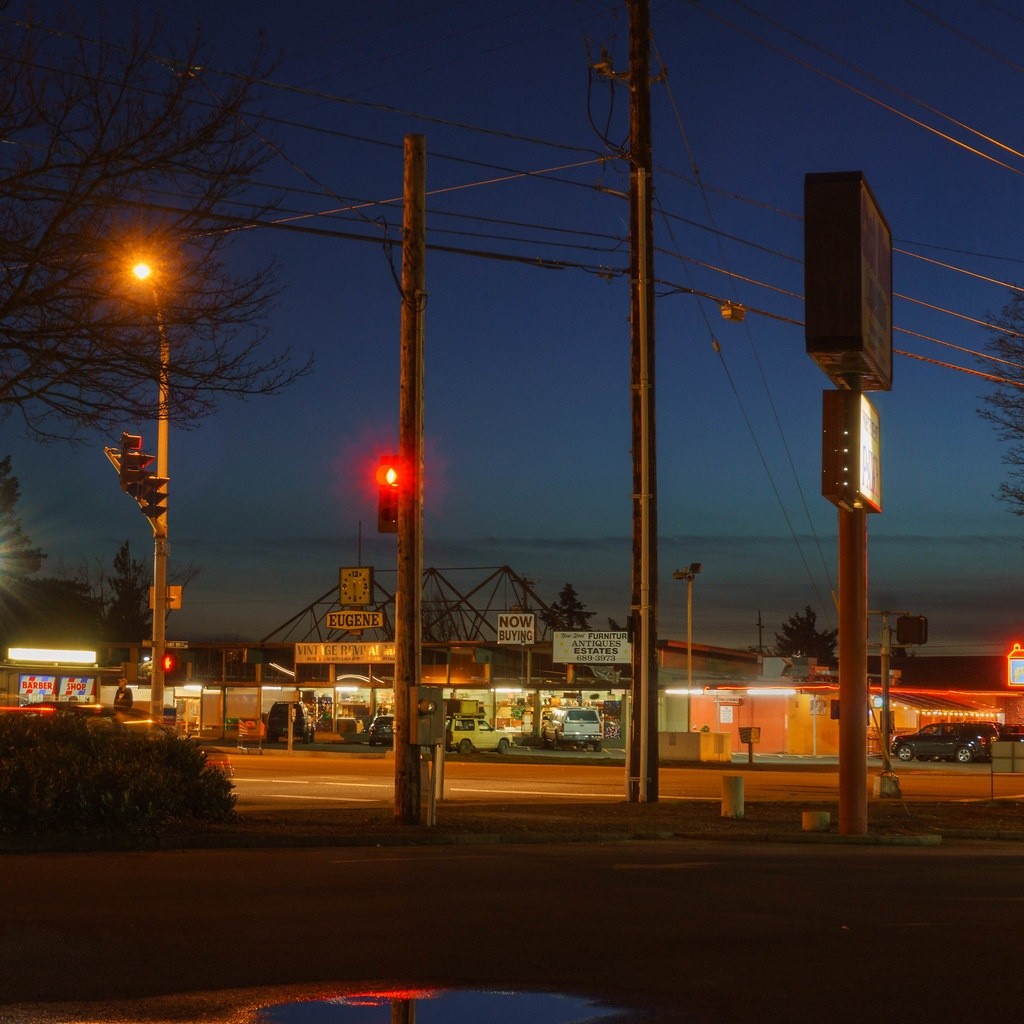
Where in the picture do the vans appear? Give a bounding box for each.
[265,700,317,744]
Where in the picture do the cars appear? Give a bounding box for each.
[444,716,516,757]
[368,714,395,746]
[1,700,235,785]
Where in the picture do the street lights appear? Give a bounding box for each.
[671,561,702,731]
[127,258,168,729]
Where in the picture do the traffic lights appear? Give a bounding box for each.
[375,455,400,533]
[118,431,171,519]
[163,651,177,672]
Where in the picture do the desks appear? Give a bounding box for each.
[659,731,732,763]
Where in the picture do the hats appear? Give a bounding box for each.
[118,678,127,683]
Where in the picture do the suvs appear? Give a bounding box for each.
[542,707,605,753]
[891,721,1024,764]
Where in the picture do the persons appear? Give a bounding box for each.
[113,678,133,712]
